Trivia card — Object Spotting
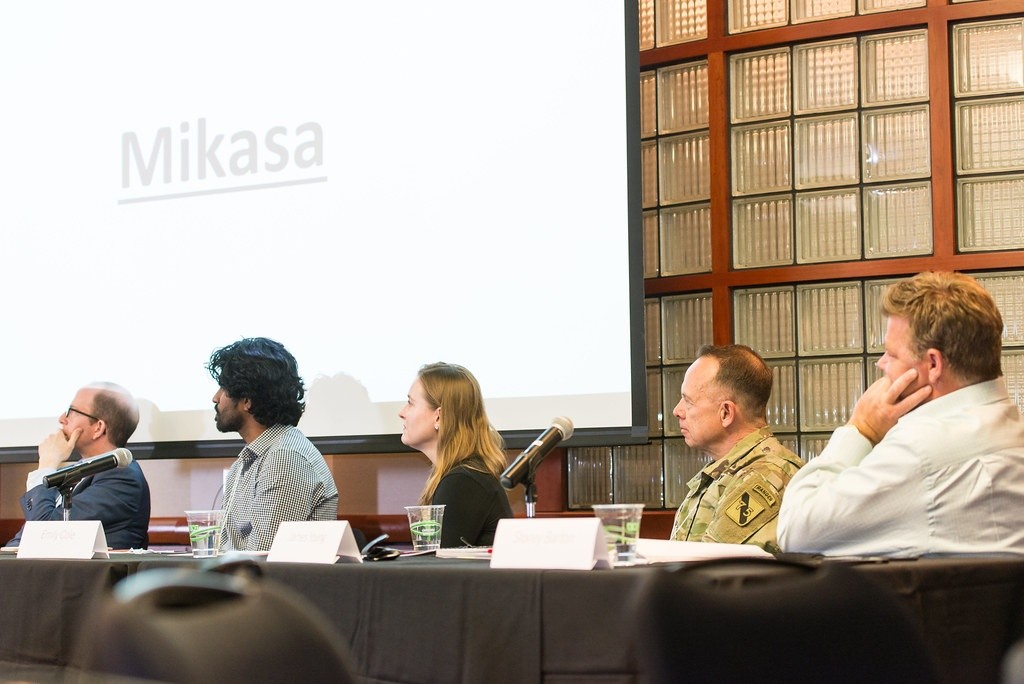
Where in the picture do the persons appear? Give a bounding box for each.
[205,336,339,550]
[5,381,150,549]
[667,344,806,551]
[777,272,1024,559]
[398,361,514,548]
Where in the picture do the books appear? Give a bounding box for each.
[436,549,492,559]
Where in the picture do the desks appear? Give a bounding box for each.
[1,543,1024,684]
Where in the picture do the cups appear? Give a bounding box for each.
[591,504,645,567]
[404,505,445,553]
[185,510,229,559]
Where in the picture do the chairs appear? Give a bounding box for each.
[63,570,358,684]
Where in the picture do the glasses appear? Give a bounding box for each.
[66,406,106,436]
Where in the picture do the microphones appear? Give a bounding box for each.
[500,416,574,491]
[42,448,132,489]
